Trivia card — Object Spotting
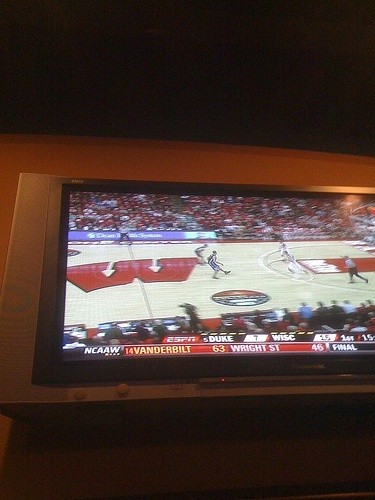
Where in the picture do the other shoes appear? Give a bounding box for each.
[348,281,354,284]
[366,279,368,283]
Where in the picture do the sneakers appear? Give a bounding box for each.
[198,263,204,265]
[212,276,219,279]
[224,271,231,275]
[204,262,207,263]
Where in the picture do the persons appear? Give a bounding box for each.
[343,255,368,284]
[61,298,375,350]
[65,189,375,246]
[276,239,292,262]
[206,250,231,279]
[194,244,208,265]
[281,250,308,275]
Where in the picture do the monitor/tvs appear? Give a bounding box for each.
[0,173,375,417]
